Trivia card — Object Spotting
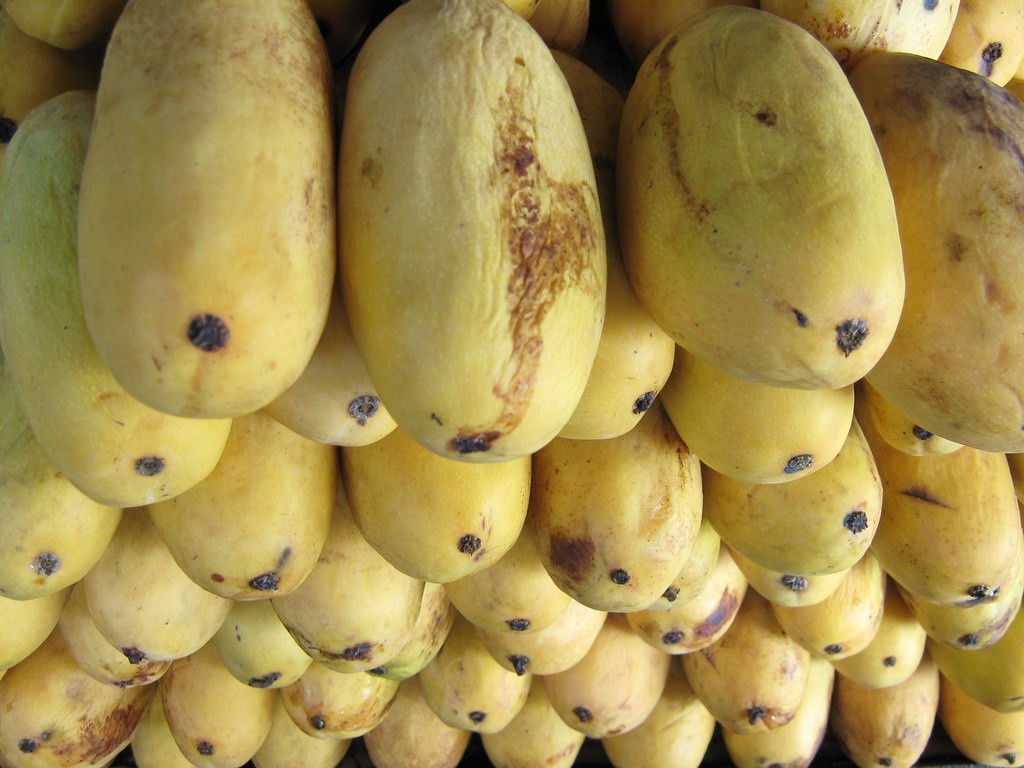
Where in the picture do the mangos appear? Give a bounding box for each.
[0,0,1024,768]
